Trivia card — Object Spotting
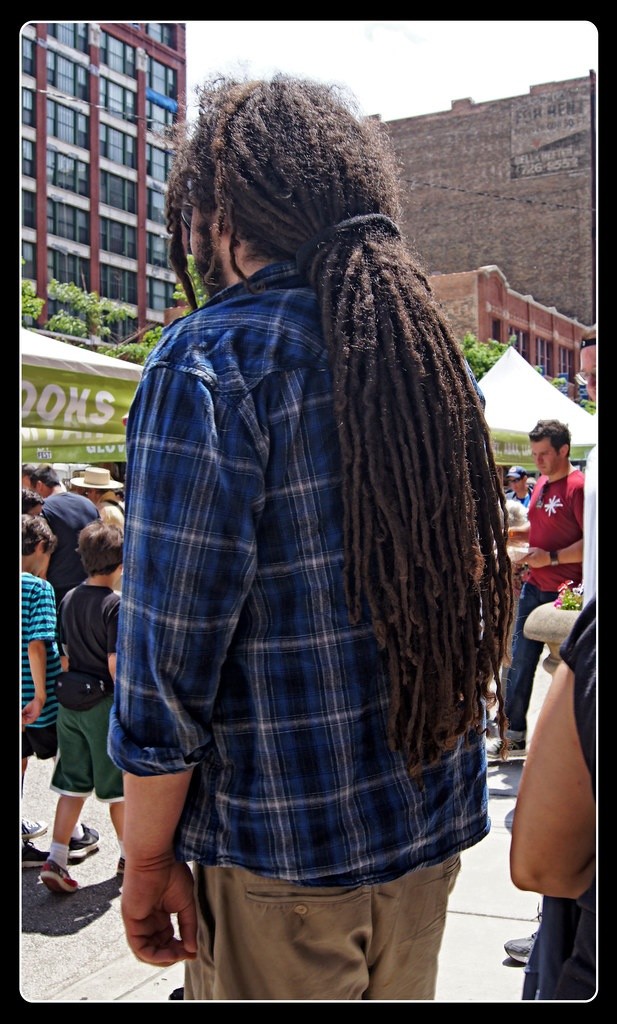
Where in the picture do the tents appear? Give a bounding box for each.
[472,346,597,466]
[20,328,147,465]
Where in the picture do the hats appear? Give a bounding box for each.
[507,465,528,479]
[71,466,124,490]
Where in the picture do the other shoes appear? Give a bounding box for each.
[39,860,79,892]
[504,930,540,963]
[22,837,51,868]
[484,736,530,758]
[115,857,126,875]
[22,817,50,836]
[68,823,101,859]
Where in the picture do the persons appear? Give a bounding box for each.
[109,79,512,1000]
[486,421,597,763]
[507,593,597,1000]
[20,463,127,895]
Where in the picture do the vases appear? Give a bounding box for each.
[523,601,583,678]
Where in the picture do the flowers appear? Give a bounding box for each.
[554,580,583,611]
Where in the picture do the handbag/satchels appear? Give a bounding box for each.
[53,671,114,710]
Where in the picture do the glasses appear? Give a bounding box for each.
[81,489,90,497]
[506,478,521,483]
